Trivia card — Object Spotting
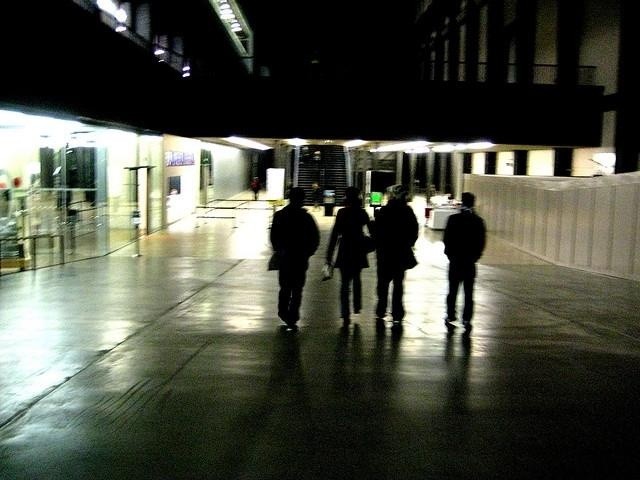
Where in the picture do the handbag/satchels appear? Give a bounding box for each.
[364,232,375,252]
[267,252,286,271]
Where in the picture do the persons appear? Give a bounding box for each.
[325,186,375,329]
[248,146,324,209]
[266,187,321,333]
[373,182,419,321]
[441,191,487,328]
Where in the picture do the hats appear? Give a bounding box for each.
[386,184,404,199]
